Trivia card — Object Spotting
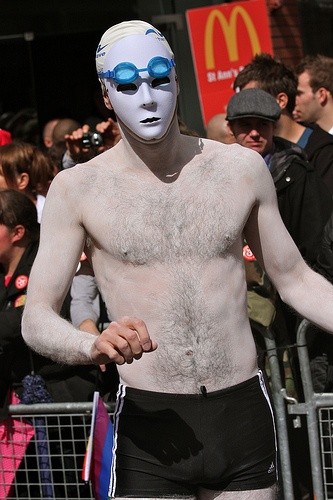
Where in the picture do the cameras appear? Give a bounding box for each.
[79,132,102,149]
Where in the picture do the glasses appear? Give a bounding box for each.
[94,56,177,84]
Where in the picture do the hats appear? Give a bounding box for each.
[225,88,282,122]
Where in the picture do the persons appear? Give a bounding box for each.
[19,21,333,500]
[225,87,332,500]
[205,112,236,145]
[294,52,333,138]
[232,52,333,226]
[0,54,121,500]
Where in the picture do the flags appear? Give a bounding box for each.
[80,391,114,500]
